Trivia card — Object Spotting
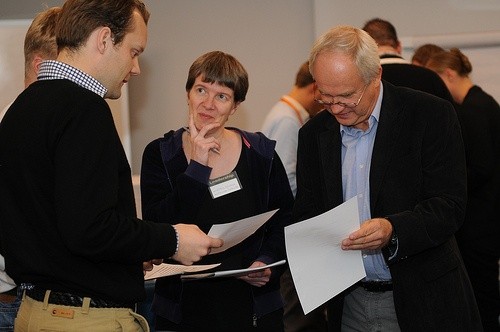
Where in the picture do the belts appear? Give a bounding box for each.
[22,282,139,313]
[357,281,394,292]
[0,294,17,303]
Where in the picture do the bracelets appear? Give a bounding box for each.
[391,231,397,245]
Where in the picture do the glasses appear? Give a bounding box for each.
[314,77,369,108]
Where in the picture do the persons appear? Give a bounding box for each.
[411,44,446,68]
[0,6,66,331]
[140,48,300,331]
[419,47,500,332]
[293,25,483,331]
[0,0,225,332]
[260,60,326,206]
[361,17,460,109]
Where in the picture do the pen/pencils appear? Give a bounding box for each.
[182,126,221,154]
